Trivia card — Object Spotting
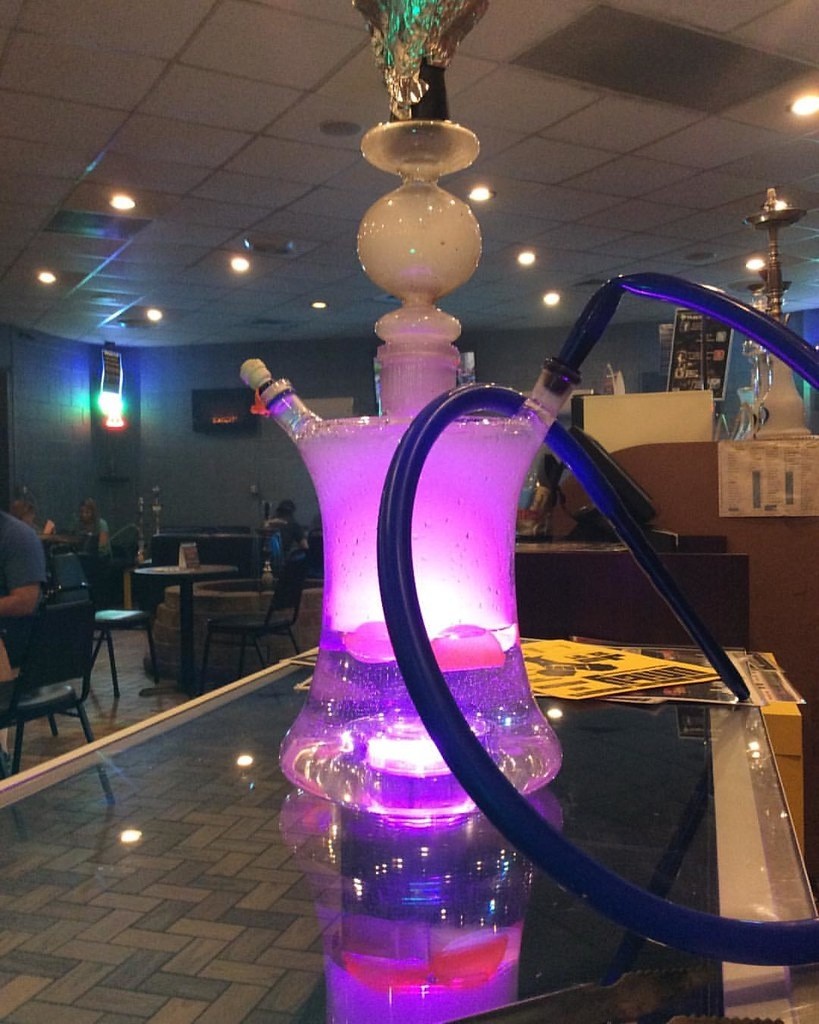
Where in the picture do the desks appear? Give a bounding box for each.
[0,634,819,1024]
[134,564,238,697]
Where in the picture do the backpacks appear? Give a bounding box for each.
[261,525,282,565]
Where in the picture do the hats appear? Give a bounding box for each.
[15,483,39,510]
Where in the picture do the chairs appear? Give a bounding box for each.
[54,553,161,697]
[199,548,309,695]
[0,583,115,835]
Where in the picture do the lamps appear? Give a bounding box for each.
[240,237,265,256]
[276,240,296,255]
[98,391,127,440]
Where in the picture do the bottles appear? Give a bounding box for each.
[676,311,712,331]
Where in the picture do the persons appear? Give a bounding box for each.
[66,497,113,560]
[0,509,47,774]
[10,500,56,536]
[260,499,310,570]
